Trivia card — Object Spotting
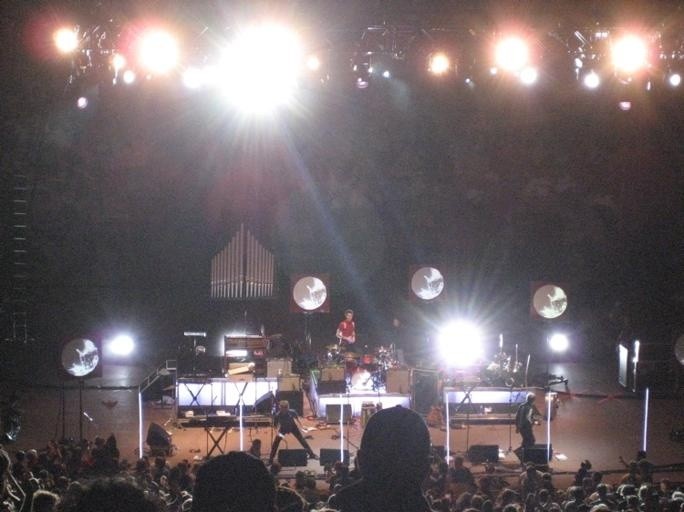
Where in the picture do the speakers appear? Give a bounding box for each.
[278,449,307,467]
[467,444,499,462]
[431,446,444,464]
[147,423,172,458]
[320,449,349,466]
[516,444,553,464]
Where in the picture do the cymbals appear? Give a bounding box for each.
[326,345,347,349]
[344,352,359,358]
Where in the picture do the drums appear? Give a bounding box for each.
[351,364,379,390]
[364,355,375,365]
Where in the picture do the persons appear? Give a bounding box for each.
[0,437,361,512]
[266,400,320,466]
[516,392,547,447]
[336,309,357,372]
[327,405,684,512]
[426,396,445,428]
[1,390,22,444]
